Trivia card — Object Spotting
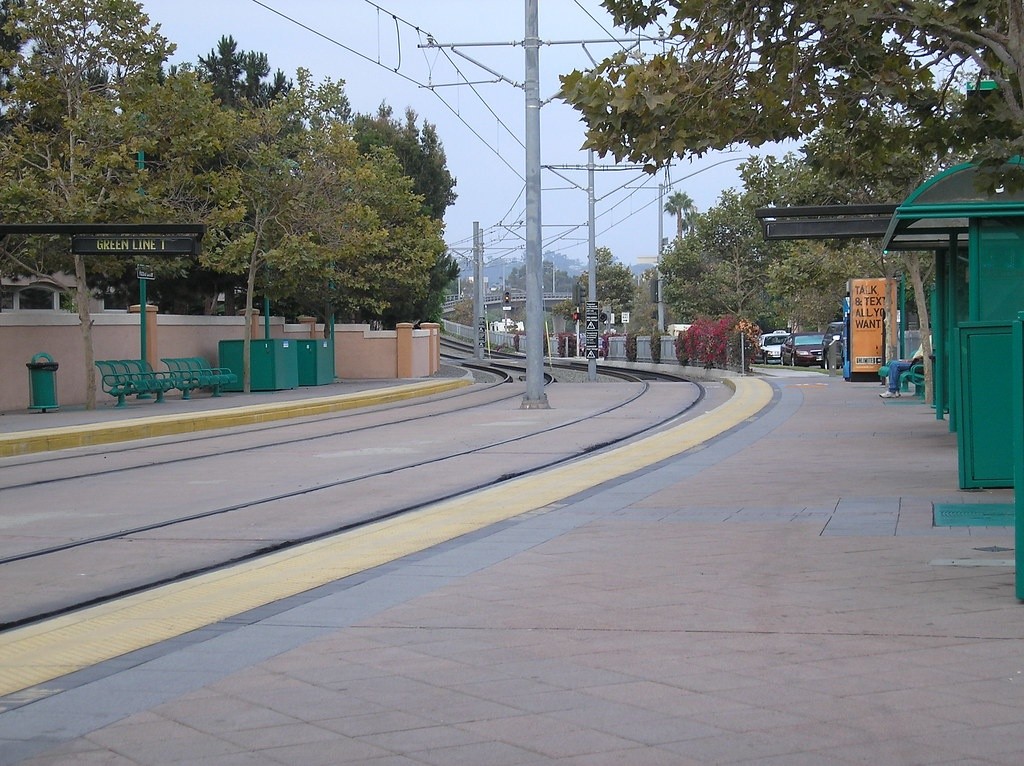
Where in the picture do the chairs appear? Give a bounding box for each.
[878,350,933,402]
[94,357,237,408]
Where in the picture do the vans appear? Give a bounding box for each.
[821,322,845,371]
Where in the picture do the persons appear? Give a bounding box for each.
[879,322,933,398]
[514,334,519,352]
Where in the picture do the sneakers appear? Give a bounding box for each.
[879,391,896,398]
[895,391,900,398]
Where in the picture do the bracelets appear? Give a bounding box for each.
[906,360,908,362]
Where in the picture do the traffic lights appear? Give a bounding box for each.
[503,290,511,306]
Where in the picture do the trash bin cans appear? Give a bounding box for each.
[26,352,60,413]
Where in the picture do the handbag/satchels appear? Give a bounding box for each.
[909,356,924,374]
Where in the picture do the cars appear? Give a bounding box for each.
[757,329,791,365]
[780,332,825,367]
